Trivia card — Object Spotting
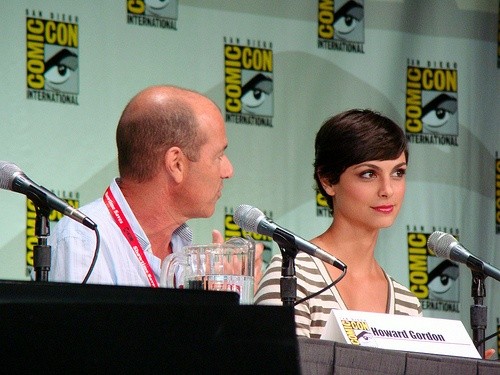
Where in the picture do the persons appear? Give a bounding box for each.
[31,85,263,300]
[255,109,423,338]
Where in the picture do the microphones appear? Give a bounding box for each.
[427,231,500,282]
[232,204,347,271]
[0,160,97,230]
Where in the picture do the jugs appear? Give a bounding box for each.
[160,235,254,307]
